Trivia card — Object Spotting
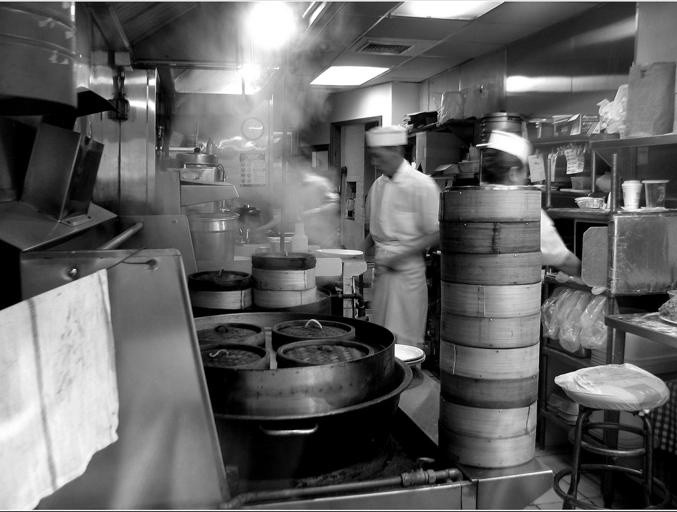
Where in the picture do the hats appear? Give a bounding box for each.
[365,125,408,148]
[486,130,533,167]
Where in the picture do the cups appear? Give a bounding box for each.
[641,180,672,208]
[620,179,643,211]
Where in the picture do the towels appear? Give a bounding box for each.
[0,271,120,512]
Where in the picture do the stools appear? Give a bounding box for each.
[554,365,671,510]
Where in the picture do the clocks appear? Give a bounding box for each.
[243,118,264,140]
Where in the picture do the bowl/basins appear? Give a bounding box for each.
[666,290,676,300]
[458,160,480,175]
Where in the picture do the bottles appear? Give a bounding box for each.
[469,144,478,160]
[291,223,310,253]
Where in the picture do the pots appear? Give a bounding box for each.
[210,355,412,475]
[233,203,262,242]
[176,147,216,167]
[187,212,239,272]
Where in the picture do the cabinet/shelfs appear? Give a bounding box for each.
[540,207,676,465]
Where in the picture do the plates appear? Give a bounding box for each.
[315,247,364,259]
[392,340,425,367]
[267,236,292,242]
[656,311,676,326]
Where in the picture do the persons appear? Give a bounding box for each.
[355,125,443,350]
[481,130,582,284]
[256,161,340,247]
